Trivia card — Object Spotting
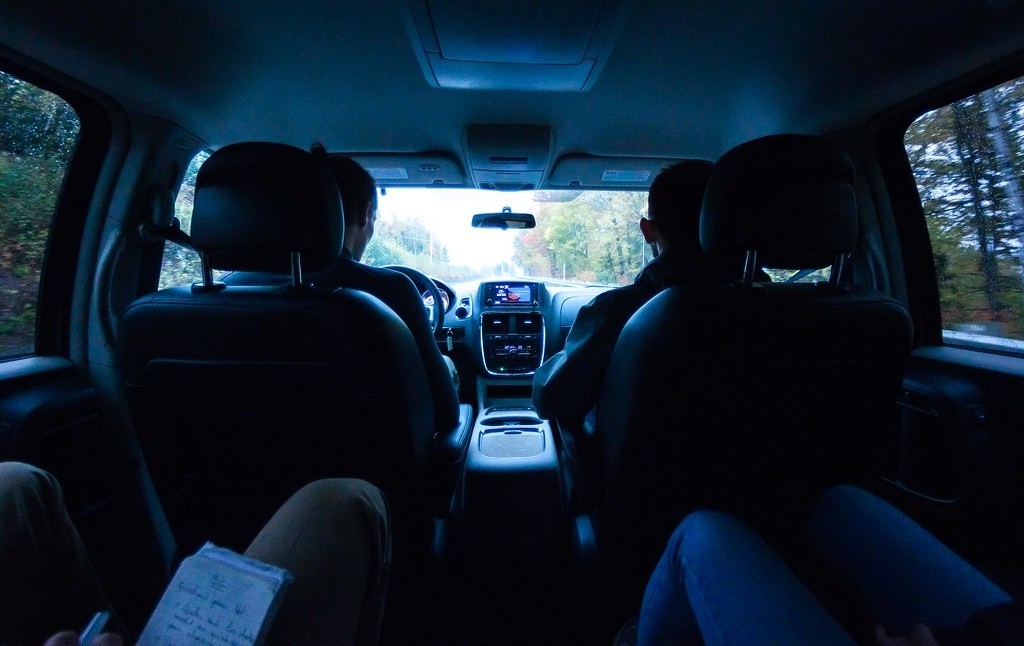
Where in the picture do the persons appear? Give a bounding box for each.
[531,159,774,480]
[218,156,460,434]
[612,475,1024,646]
[0,461,391,646]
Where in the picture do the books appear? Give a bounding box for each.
[135,541,295,646]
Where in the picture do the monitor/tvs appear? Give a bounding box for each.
[493,285,531,304]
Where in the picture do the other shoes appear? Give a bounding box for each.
[614,616,639,646]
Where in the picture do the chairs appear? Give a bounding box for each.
[555,132,916,591]
[115,141,474,592]
